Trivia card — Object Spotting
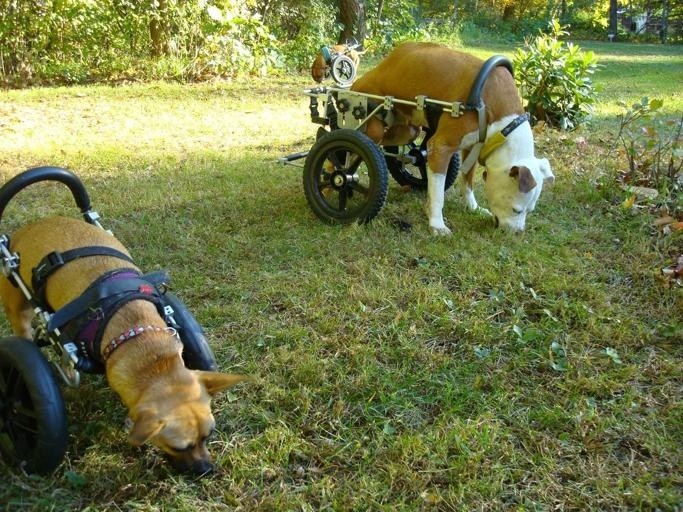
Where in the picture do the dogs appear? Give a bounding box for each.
[311,41,556,240]
[0,215,253,481]
